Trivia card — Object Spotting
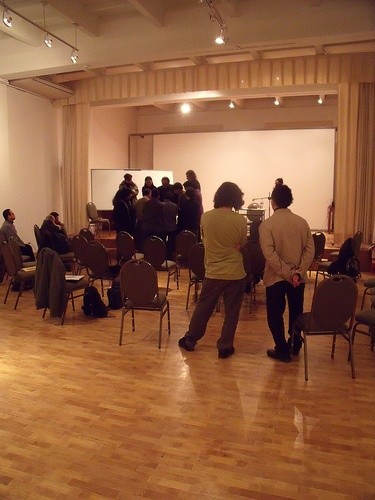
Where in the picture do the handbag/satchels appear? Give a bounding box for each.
[346,257,361,278]
[12,277,34,291]
[106,287,123,309]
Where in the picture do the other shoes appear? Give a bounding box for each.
[268,348,291,362]
[219,347,235,359]
[179,338,196,351]
[289,342,300,354]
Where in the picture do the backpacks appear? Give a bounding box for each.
[82,285,108,318]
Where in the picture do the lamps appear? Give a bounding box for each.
[71,51,78,63]
[45,33,52,48]
[3,5,12,27]
[216,28,225,44]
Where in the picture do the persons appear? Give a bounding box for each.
[113,170,203,268]
[245,219,262,250]
[257,185,316,362]
[39,211,73,255]
[178,182,247,360]
[0,209,36,263]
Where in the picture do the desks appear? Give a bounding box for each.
[238,208,266,234]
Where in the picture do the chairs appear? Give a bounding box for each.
[0,202,375,382]
